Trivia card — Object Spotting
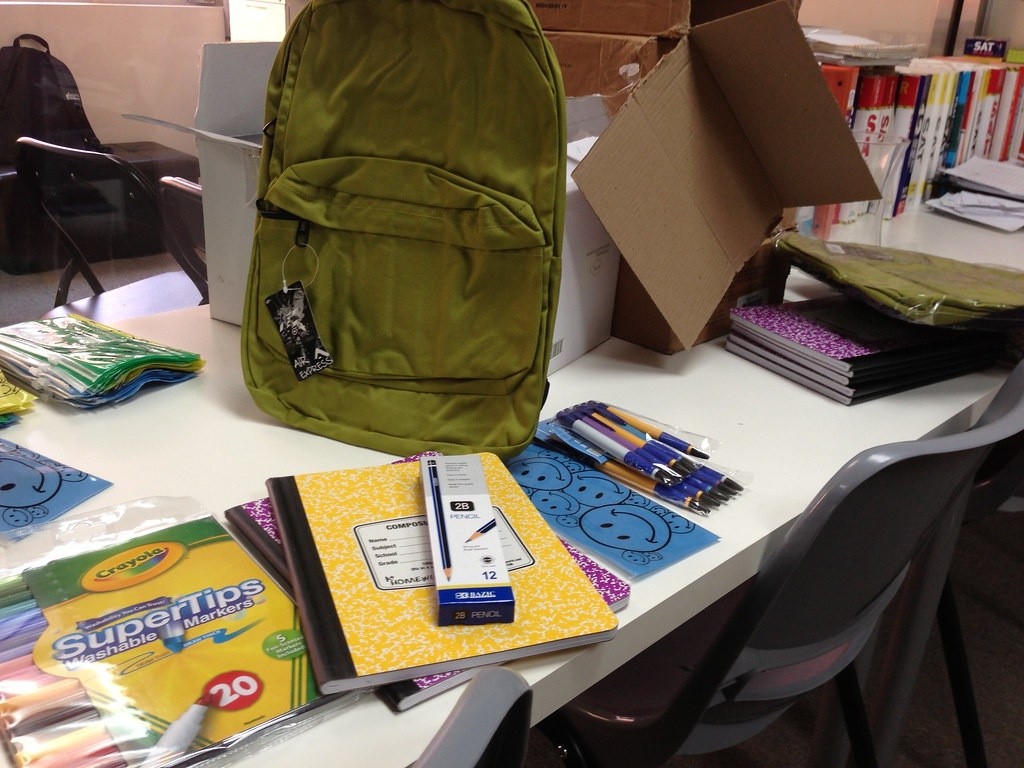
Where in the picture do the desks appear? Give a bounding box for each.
[0,249,1024,768]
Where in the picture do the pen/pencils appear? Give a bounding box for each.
[581,403,700,472]
[557,411,673,486]
[464,518,497,543]
[580,452,710,514]
[565,407,685,483]
[427,460,452,583]
[587,400,710,461]
[574,404,690,476]
[666,464,744,506]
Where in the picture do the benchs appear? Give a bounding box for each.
[0,140,199,276]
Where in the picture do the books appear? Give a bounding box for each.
[798,37,1024,236]
[223,450,629,714]
[722,291,1024,406]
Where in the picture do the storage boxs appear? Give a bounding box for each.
[119,0,884,372]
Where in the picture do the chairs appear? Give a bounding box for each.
[542,355,1024,768]
[11,135,203,324]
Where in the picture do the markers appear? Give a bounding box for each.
[137,693,214,768]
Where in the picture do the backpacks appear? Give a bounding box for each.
[0,34,113,167]
[240,0,567,459]
